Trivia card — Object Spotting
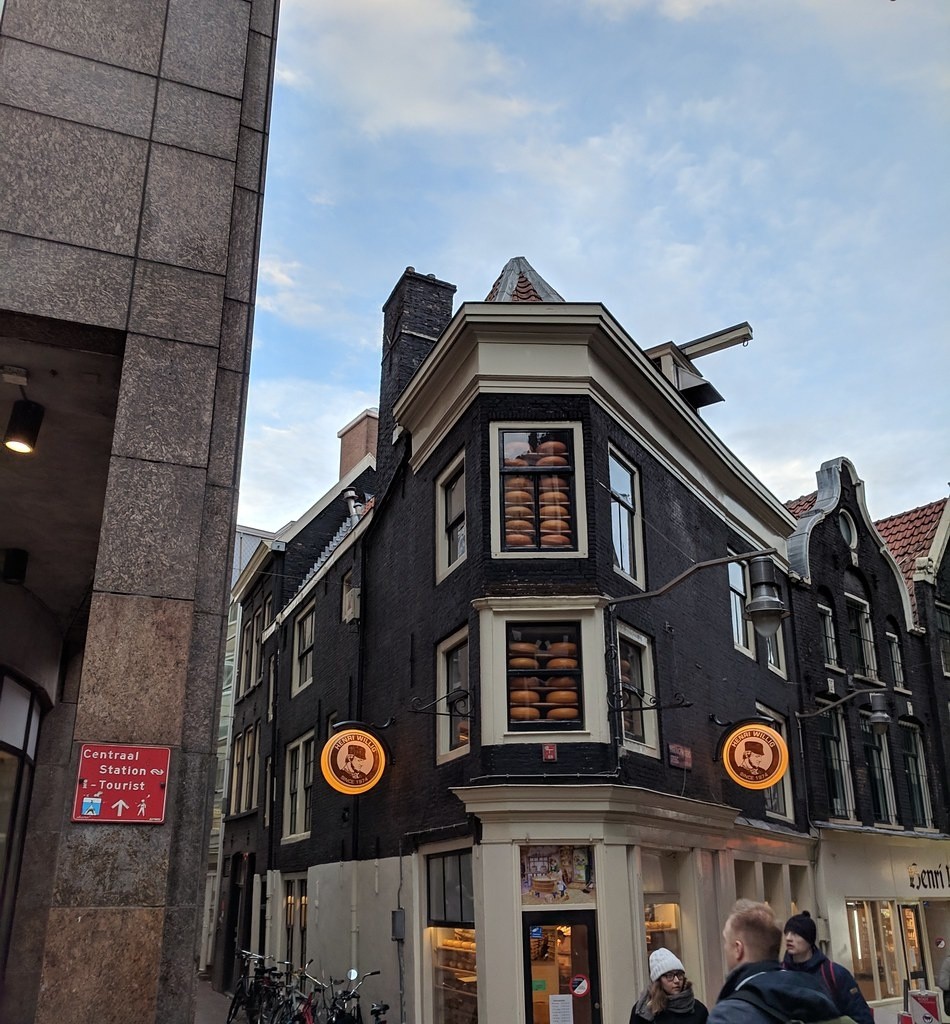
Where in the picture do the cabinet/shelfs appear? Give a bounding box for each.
[430,928,478,1024]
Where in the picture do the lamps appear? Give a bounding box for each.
[742,557,790,638]
[869,694,891,735]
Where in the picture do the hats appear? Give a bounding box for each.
[649,947,686,983]
[784,910,817,946]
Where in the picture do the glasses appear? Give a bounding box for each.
[663,971,684,982]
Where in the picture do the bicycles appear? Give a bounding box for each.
[225,947,390,1024]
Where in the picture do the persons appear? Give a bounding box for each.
[781,911,876,1024]
[707,898,840,1024]
[627,948,709,1023]
